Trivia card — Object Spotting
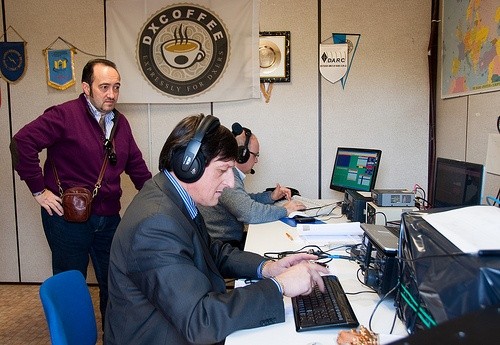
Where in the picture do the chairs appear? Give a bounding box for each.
[39,269,98,345]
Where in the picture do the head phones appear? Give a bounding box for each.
[235,127,251,164]
[171,115,220,184]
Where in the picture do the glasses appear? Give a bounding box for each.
[249,151,260,158]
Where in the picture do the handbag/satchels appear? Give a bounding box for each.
[61,187,92,223]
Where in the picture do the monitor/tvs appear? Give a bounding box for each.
[330,147,382,207]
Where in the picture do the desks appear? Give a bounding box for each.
[225,196,404,345]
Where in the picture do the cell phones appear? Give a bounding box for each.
[296,217,316,223]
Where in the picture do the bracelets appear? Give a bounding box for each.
[32,188,47,196]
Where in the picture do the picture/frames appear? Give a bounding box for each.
[259,31,291,83]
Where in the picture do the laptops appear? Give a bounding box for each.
[360,157,484,255]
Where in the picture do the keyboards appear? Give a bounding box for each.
[273,195,325,211]
[291,275,358,332]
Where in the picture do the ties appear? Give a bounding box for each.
[98,114,107,135]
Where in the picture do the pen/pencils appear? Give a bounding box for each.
[327,254,345,258]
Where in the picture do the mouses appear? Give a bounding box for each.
[288,210,309,219]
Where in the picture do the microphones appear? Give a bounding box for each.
[250,169,256,174]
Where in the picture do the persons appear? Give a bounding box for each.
[10,60,152,336]
[103,113,329,345]
[196,122,307,248]
